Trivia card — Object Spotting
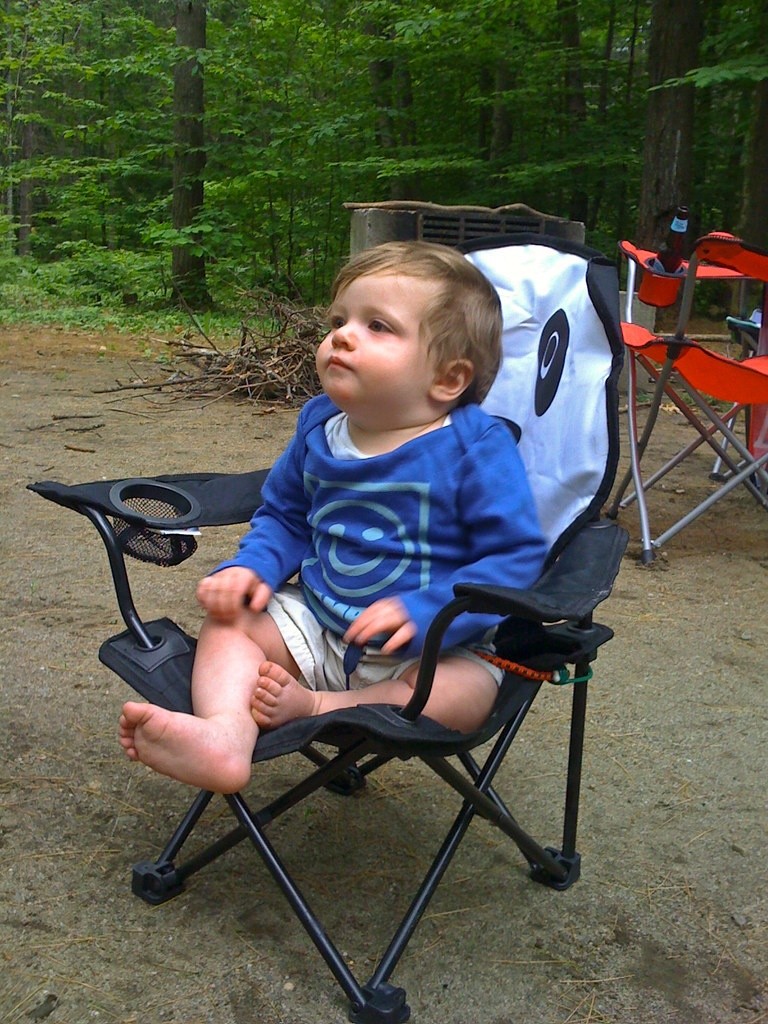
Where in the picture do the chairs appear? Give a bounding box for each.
[26,232,633,1024]
[618,231,768,562]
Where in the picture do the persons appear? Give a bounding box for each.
[118,239,546,795]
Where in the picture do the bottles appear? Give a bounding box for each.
[653,206,689,274]
